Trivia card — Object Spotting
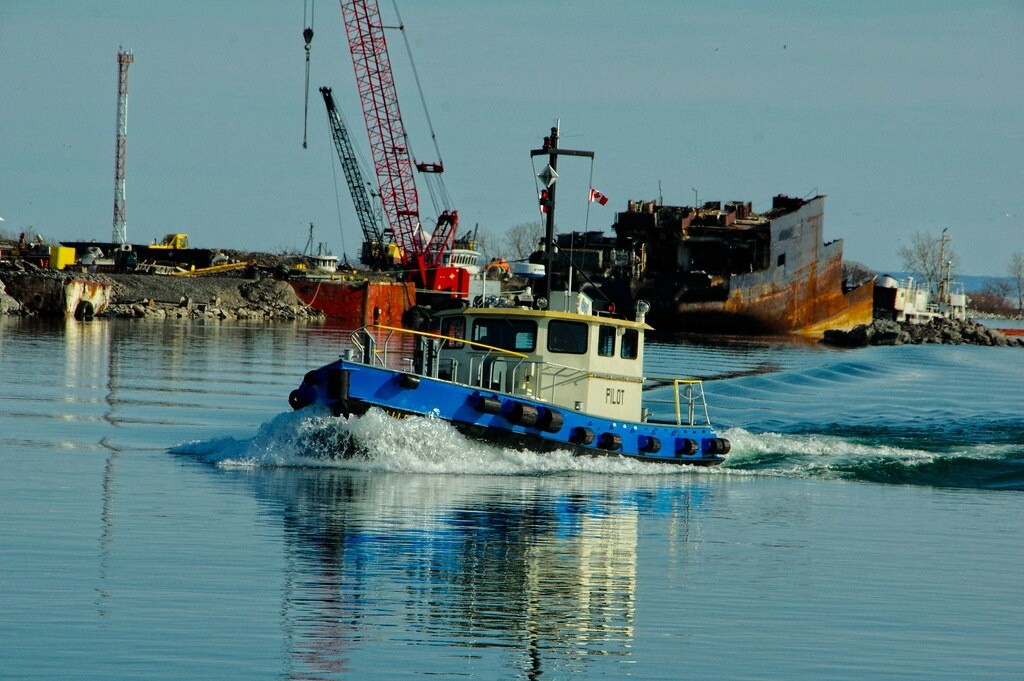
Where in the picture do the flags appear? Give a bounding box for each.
[589,189,608,207]
[538,189,548,214]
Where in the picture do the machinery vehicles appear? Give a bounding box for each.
[301,1,470,297]
[317,83,404,272]
[149,234,189,249]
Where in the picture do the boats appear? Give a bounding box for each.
[287,117,731,465]
[286,248,503,325]
